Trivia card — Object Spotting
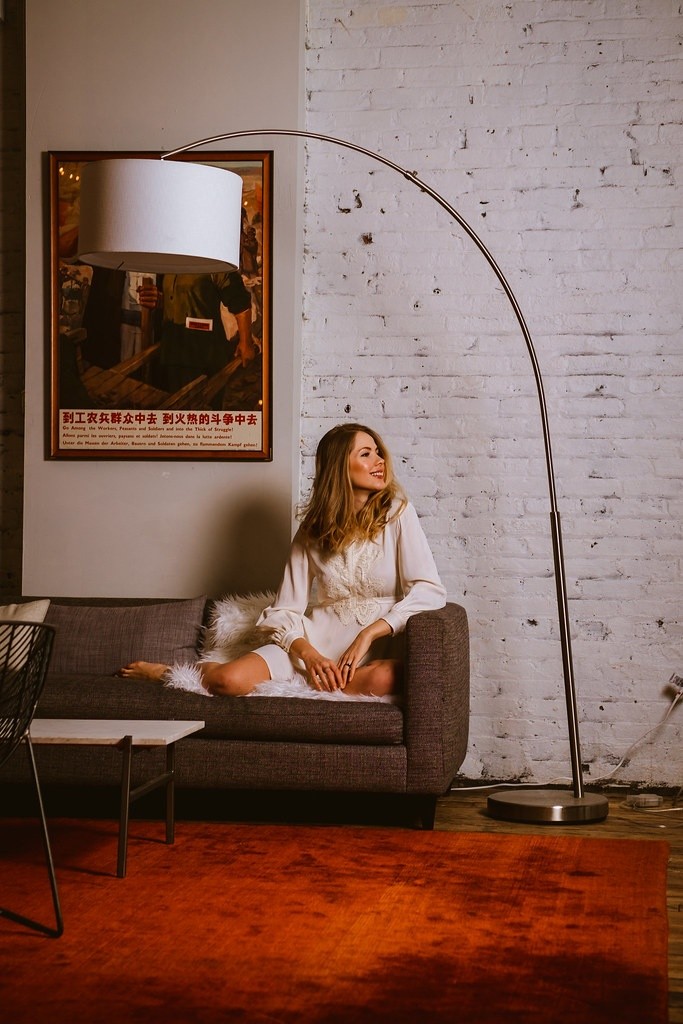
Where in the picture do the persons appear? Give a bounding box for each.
[121,423,446,697]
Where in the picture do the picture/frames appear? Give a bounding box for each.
[45,148,274,462]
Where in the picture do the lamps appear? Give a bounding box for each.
[75,129,610,822]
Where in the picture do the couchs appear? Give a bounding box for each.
[0,596,470,830]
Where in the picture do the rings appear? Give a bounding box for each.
[345,663,351,668]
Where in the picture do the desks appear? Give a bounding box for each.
[0,718,205,878]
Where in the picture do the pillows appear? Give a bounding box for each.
[0,598,50,670]
[37,594,208,674]
